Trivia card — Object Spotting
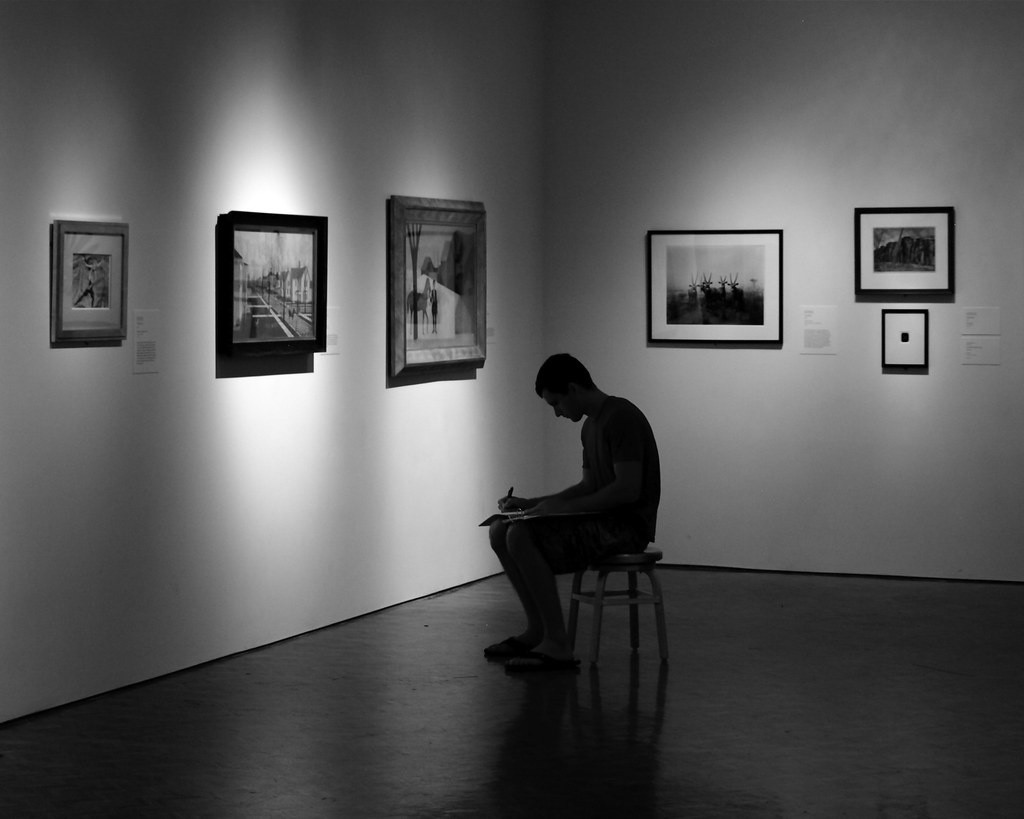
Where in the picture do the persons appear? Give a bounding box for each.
[484,351,662,671]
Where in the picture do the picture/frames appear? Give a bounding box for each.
[648,229,783,345]
[882,309,929,369]
[855,206,956,295]
[390,195,488,378]
[220,211,328,355]
[51,219,129,343]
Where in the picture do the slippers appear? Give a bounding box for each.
[505,651,582,673]
[484,637,534,657]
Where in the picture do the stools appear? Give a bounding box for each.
[565,545,670,663]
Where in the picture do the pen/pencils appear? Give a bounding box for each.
[507,486,514,498]
